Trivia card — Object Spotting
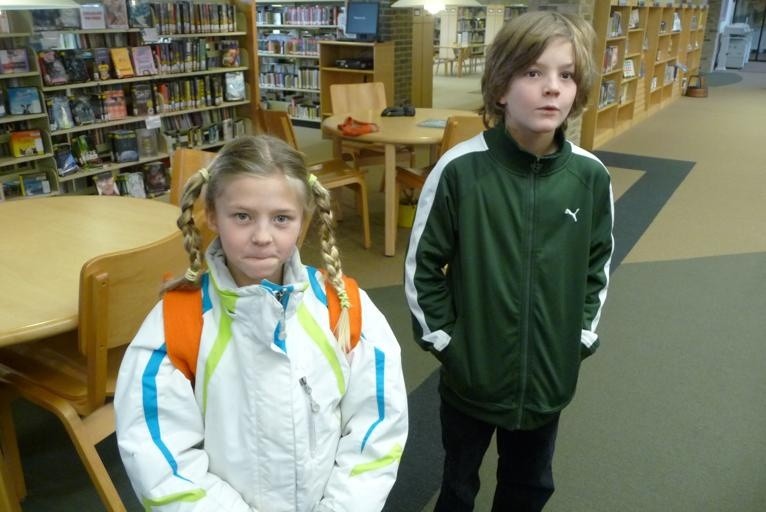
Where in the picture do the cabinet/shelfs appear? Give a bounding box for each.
[318,39,395,139]
[256,0,345,122]
[0,0,260,196]
[435,3,558,59]
[560,2,709,153]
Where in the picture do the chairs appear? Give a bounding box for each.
[262,82,497,258]
[170,148,221,203]
[0,213,215,512]
[434,42,490,77]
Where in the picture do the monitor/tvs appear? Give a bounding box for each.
[346,2,380,38]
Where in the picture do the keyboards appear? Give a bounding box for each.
[337,38,374,42]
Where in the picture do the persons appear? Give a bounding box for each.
[402,8,617,512]
[111,135,410,512]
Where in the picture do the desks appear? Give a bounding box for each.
[2,193,185,511]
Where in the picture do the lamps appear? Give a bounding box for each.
[390,0,484,15]
